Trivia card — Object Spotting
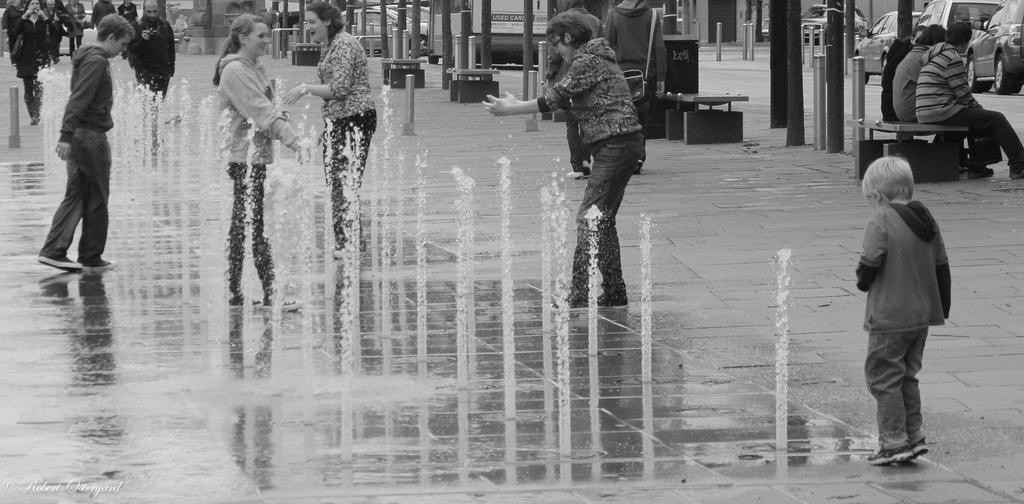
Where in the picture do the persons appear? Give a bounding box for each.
[2,1,24,66]
[548,54,596,182]
[212,13,313,316]
[602,1,668,177]
[117,0,137,33]
[10,0,56,128]
[279,1,379,264]
[892,24,972,169]
[62,1,88,59]
[43,0,62,65]
[123,1,177,105]
[89,0,116,31]
[480,7,647,310]
[855,155,954,468]
[37,12,137,274]
[913,18,1023,182]
[881,25,929,122]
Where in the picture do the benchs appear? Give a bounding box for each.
[656,92,749,145]
[847,119,969,183]
[382,58,427,88]
[291,42,321,66]
[446,68,499,104]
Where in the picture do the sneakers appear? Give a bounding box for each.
[583,161,590,175]
[896,440,928,463]
[228,296,262,309]
[868,445,912,465]
[1010,168,1024,179]
[967,168,993,179]
[264,297,302,312]
[567,170,582,179]
[633,160,643,174]
[39,254,83,271]
[77,259,118,270]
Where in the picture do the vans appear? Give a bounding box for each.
[915,0,999,43]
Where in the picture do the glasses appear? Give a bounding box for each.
[553,38,562,47]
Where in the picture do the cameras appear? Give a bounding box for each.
[147,27,157,35]
[30,8,39,15]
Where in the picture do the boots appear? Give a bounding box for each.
[31,97,41,124]
[25,98,39,125]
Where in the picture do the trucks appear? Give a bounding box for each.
[427,0,555,68]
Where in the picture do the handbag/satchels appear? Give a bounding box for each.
[622,8,656,102]
[967,105,1003,164]
[78,14,90,29]
[10,32,23,64]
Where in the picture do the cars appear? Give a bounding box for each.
[855,12,922,84]
[966,0,1024,95]
[341,9,428,56]
[373,5,430,19]
[801,4,869,45]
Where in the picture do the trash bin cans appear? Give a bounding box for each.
[664,35,699,95]
